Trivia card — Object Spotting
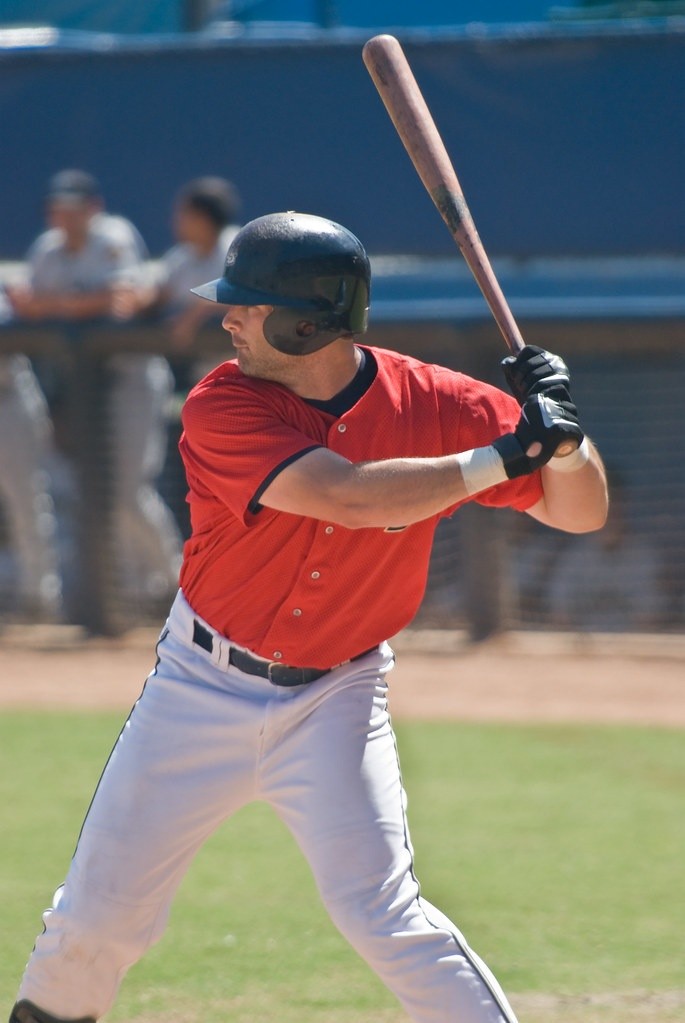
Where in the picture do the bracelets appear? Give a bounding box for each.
[546,435,589,473]
[459,446,509,495]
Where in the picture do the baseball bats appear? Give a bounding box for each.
[360,31,579,460]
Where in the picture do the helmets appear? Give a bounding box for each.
[189,211,371,357]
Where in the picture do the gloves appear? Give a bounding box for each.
[492,384,584,481]
[499,344,572,408]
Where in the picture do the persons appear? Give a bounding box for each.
[116,177,258,545]
[10,210,610,1023]
[0,170,185,641]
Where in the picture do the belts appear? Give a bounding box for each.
[193,618,382,688]
